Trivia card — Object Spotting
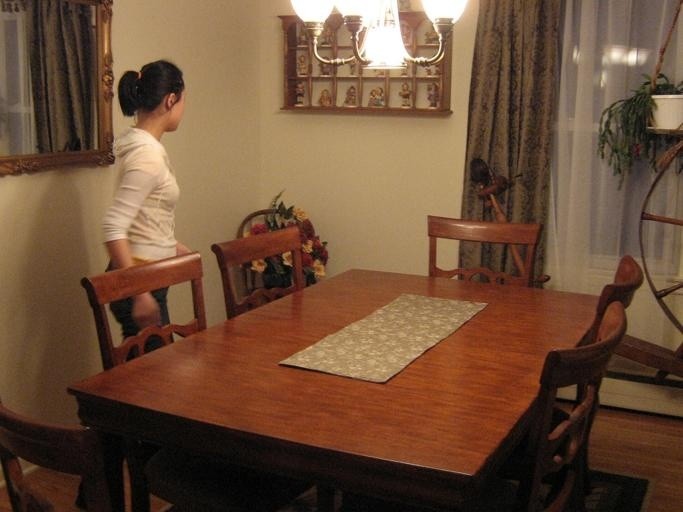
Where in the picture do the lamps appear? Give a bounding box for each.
[290,0,468,70]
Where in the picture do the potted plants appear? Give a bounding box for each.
[597,75,683,185]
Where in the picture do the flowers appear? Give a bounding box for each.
[241,187,328,281]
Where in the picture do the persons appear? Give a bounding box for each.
[100,58,192,364]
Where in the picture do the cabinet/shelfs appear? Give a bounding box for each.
[279,14,454,118]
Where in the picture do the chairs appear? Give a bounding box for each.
[472,299,627,511]
[584,253,644,344]
[428,215,543,287]
[269,463,460,511]
[1,406,175,512]
[211,226,304,319]
[81,252,316,510]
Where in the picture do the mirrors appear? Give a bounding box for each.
[0,1,115,176]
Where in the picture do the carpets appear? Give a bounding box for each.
[162,463,651,511]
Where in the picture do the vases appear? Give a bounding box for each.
[263,266,292,288]
